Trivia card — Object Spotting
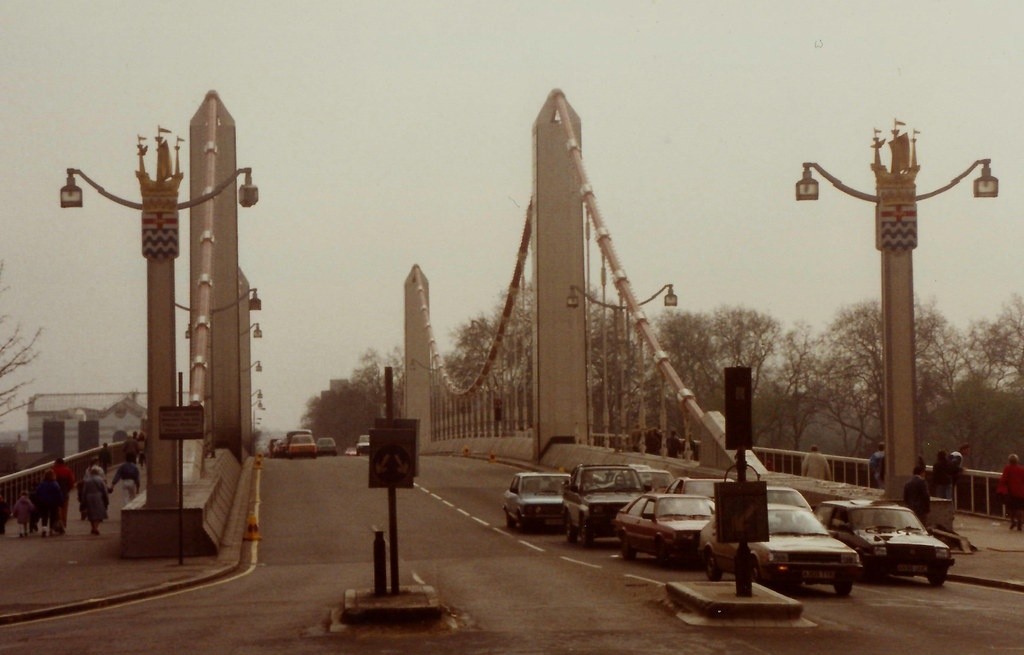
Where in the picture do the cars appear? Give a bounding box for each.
[265,427,371,458]
[501,473,565,532]
[665,476,735,500]
[700,504,862,598]
[616,493,718,570]
[765,486,813,513]
[814,499,957,588]
[631,466,676,494]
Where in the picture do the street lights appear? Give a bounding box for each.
[795,154,999,495]
[565,283,679,449]
[208,283,263,456]
[58,164,259,503]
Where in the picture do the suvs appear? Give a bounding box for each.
[562,463,644,548]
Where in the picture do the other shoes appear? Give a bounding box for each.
[92,528,100,535]
[19,527,65,538]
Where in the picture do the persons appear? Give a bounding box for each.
[871,443,963,499]
[801,446,831,481]
[904,464,930,528]
[634,424,696,460]
[0,430,145,536]
[998,454,1024,531]
